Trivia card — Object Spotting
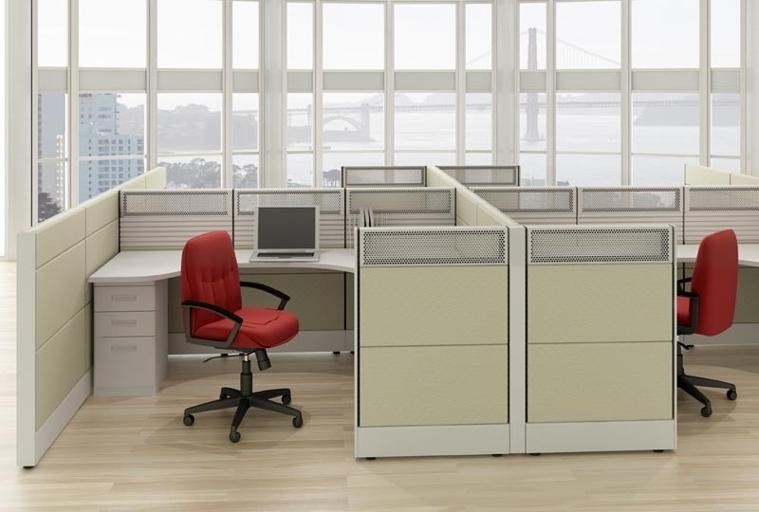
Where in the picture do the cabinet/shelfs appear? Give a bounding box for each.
[89,283,167,400]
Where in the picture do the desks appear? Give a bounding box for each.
[87,244,357,290]
[677,240,757,272]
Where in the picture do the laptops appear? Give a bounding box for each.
[249,204,320,263]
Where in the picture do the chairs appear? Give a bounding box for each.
[677,227,745,424]
[166,229,301,445]
